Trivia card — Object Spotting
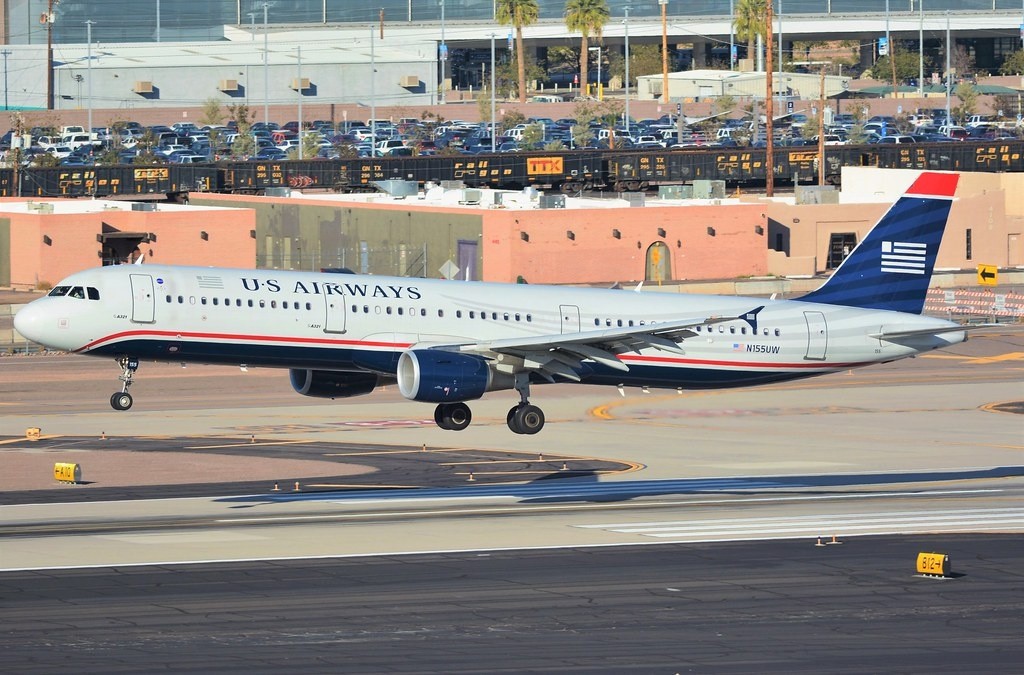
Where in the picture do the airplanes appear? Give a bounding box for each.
[11,167,970,436]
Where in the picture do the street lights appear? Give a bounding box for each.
[621,4,635,132]
[588,47,603,102]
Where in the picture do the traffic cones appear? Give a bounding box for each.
[574,74,580,84]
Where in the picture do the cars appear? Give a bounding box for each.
[939,73,977,87]
[899,76,932,88]
[0,105,1023,160]
